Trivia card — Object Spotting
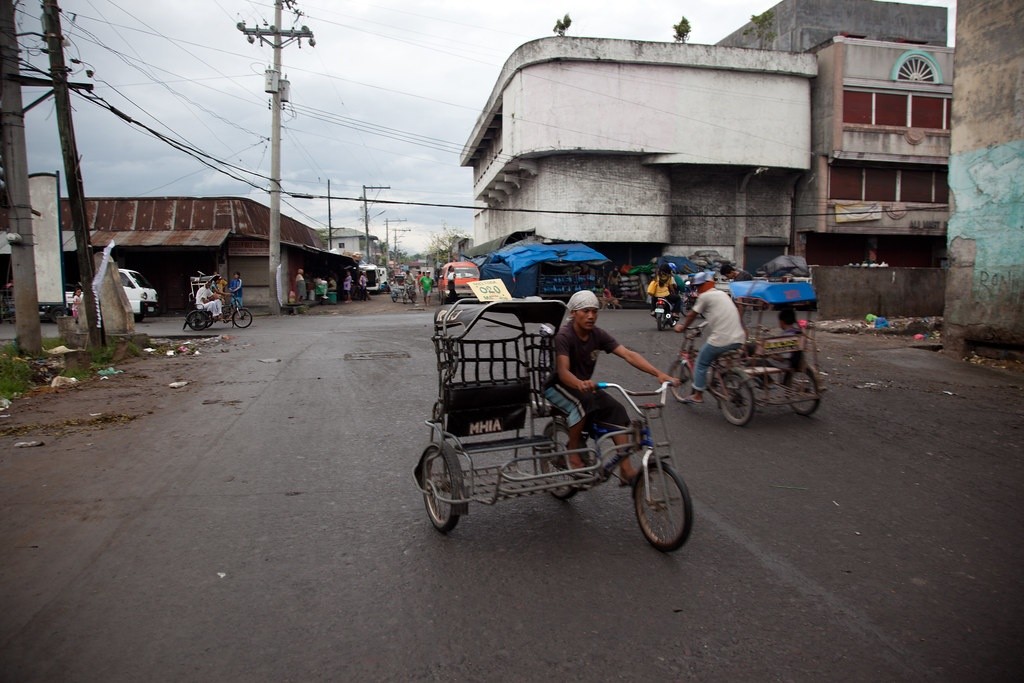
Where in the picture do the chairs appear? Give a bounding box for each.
[739,335,800,400]
[442,381,558,495]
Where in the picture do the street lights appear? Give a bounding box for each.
[0,28,66,358]
[364,208,387,264]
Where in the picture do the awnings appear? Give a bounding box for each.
[462,227,536,261]
[0,231,12,254]
[88,228,232,247]
[62,230,98,251]
[249,233,362,262]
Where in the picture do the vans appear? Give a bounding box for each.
[358,264,405,295]
[437,261,481,306]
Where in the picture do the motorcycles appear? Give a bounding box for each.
[650,281,691,332]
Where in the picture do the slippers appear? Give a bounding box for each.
[684,394,705,404]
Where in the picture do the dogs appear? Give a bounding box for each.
[597,297,623,310]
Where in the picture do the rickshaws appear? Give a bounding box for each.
[747,254,820,336]
[667,275,828,428]
[386,269,418,305]
[181,270,254,331]
[410,296,696,554]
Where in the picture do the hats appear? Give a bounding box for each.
[690,272,714,285]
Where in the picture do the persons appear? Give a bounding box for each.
[358,271,369,302]
[540,290,682,488]
[210,270,244,319]
[72,288,82,316]
[778,309,801,336]
[401,271,416,298]
[344,271,353,304]
[674,271,748,404]
[650,263,685,321]
[295,268,307,304]
[327,277,337,291]
[417,270,434,307]
[195,280,225,321]
[446,266,459,304]
[600,266,623,312]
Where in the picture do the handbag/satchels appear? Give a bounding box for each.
[647,280,658,294]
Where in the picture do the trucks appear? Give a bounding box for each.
[38,267,160,325]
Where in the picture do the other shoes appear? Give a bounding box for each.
[561,458,587,473]
[650,312,655,315]
[619,471,653,487]
[672,315,680,318]
